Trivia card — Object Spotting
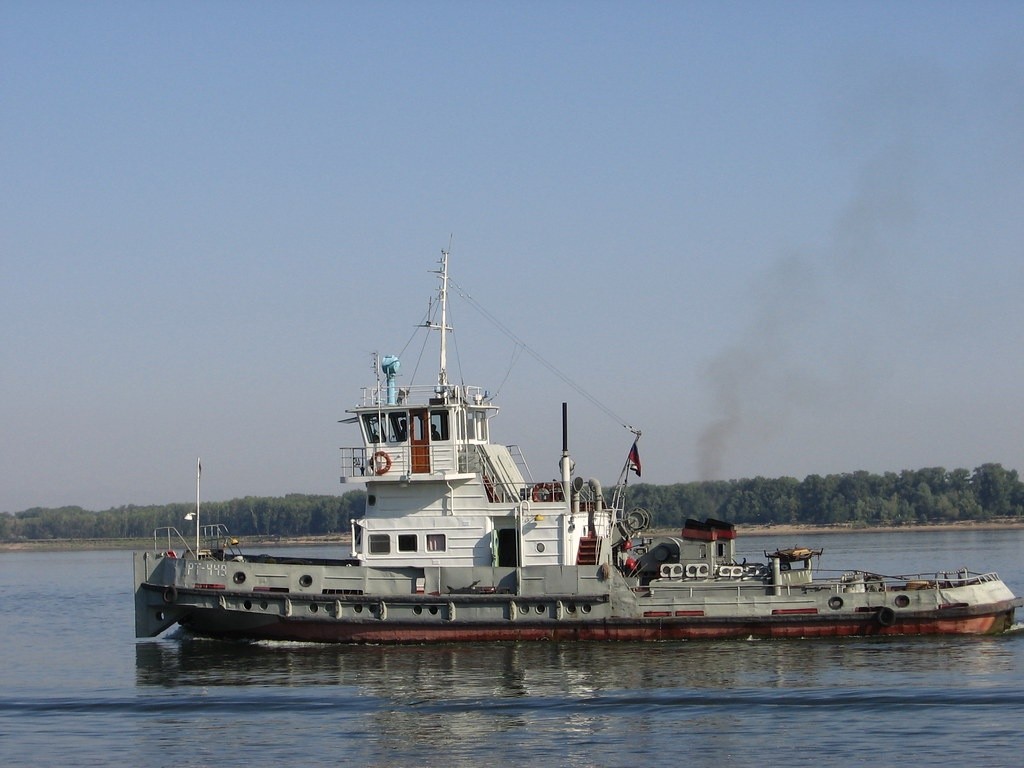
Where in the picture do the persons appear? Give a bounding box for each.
[400,419,406,439]
[432,424,440,440]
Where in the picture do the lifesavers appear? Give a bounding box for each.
[370,451,391,474]
[532,482,556,502]
[163,585,178,603]
[876,607,896,626]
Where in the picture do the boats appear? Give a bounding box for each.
[134,233,1024,642]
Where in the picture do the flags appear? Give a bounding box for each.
[628,443,642,477]
